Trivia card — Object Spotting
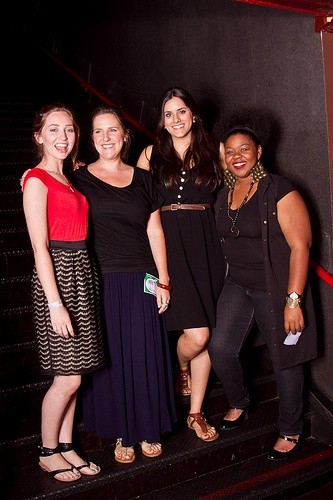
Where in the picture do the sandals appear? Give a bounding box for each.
[38,442,101,482]
[187,412,219,442]
[139,440,162,457]
[177,367,193,396]
[114,438,135,463]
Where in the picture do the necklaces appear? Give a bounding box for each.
[56,174,74,192]
[226,177,254,237]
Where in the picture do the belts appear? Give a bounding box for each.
[161,204,213,211]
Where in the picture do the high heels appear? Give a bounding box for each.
[219,406,251,430]
[266,433,303,460]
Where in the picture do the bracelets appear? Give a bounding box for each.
[287,298,300,308]
[157,282,171,290]
[48,300,63,308]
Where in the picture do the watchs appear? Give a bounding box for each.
[286,292,303,300]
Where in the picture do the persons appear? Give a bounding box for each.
[20,108,179,463]
[22,105,101,483]
[72,87,228,442]
[207,126,317,461]
